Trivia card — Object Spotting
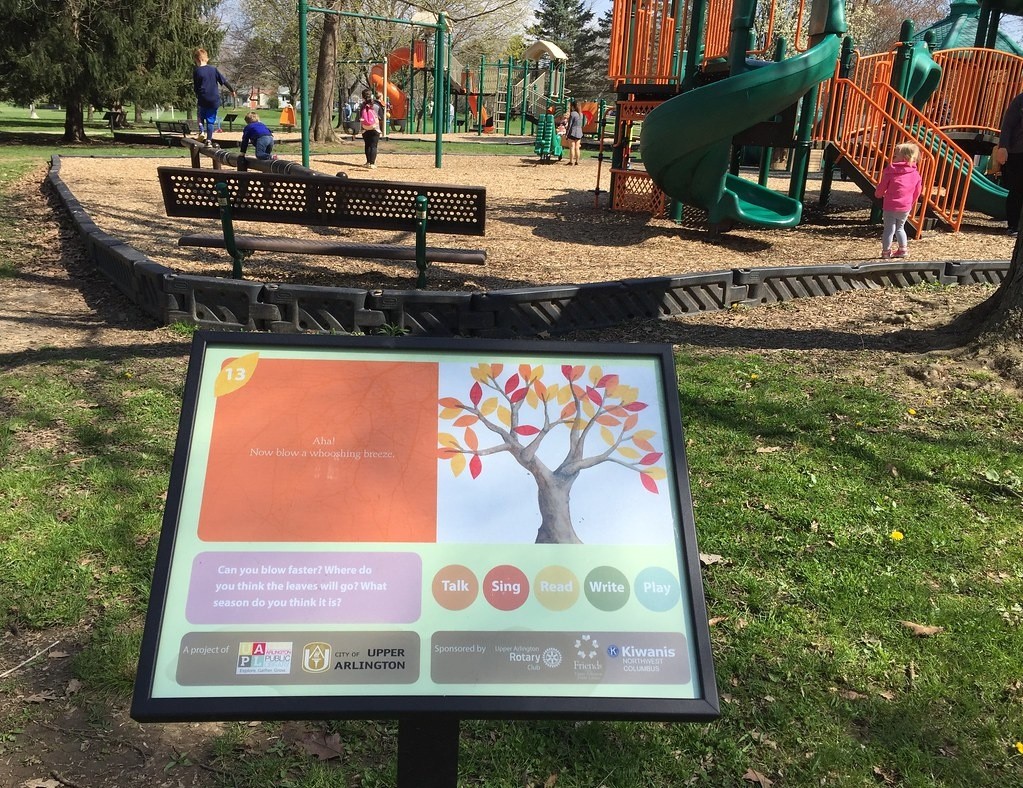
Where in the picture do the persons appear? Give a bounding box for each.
[450,101,454,127]
[239,112,277,160]
[193,49,237,148]
[360,89,383,169]
[565,101,583,165]
[997,92,1023,238]
[875,143,922,259]
[397,80,403,90]
[344,101,352,122]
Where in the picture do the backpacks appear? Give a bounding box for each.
[359,109,382,134]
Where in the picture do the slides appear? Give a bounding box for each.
[652,33,839,228]
[905,124,1009,219]
[369,47,411,119]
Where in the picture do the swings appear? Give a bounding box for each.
[341,13,361,135]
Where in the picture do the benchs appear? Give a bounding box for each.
[155,121,197,148]
[156,165,488,288]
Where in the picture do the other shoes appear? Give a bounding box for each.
[364,163,377,169]
[198,132,205,141]
[566,162,572,166]
[204,140,212,147]
[882,249,896,259]
[575,162,578,165]
[893,248,910,258]
